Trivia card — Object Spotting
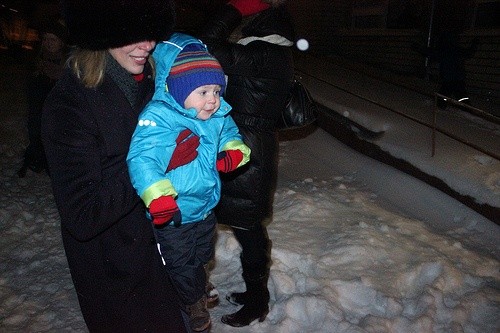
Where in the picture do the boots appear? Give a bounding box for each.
[221,240,272,327]
[182,264,219,331]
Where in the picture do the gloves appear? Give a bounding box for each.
[216,149,243,173]
[150,196,181,227]
[228,0,271,16]
[166,129,200,172]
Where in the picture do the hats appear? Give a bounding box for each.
[42,23,64,42]
[70,0,161,49]
[167,44,226,109]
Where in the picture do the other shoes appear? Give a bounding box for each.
[24,143,43,153]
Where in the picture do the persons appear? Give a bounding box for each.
[26,22,73,181]
[198,0,290,327]
[126,32,251,331]
[42,19,191,332]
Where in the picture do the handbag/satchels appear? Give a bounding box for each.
[279,71,319,140]
